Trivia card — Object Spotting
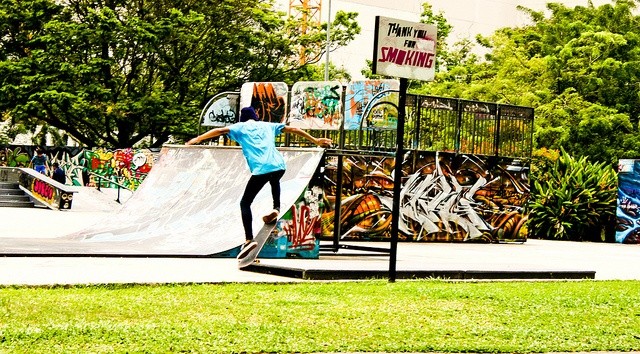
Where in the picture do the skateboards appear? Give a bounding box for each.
[238,222,277,268]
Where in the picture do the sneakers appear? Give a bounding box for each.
[263,211,278,223]
[237,241,257,260]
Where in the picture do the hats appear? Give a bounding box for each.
[240,107,258,122]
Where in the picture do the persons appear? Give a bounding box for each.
[28,149,48,175]
[185,107,334,258]
[51,162,65,184]
